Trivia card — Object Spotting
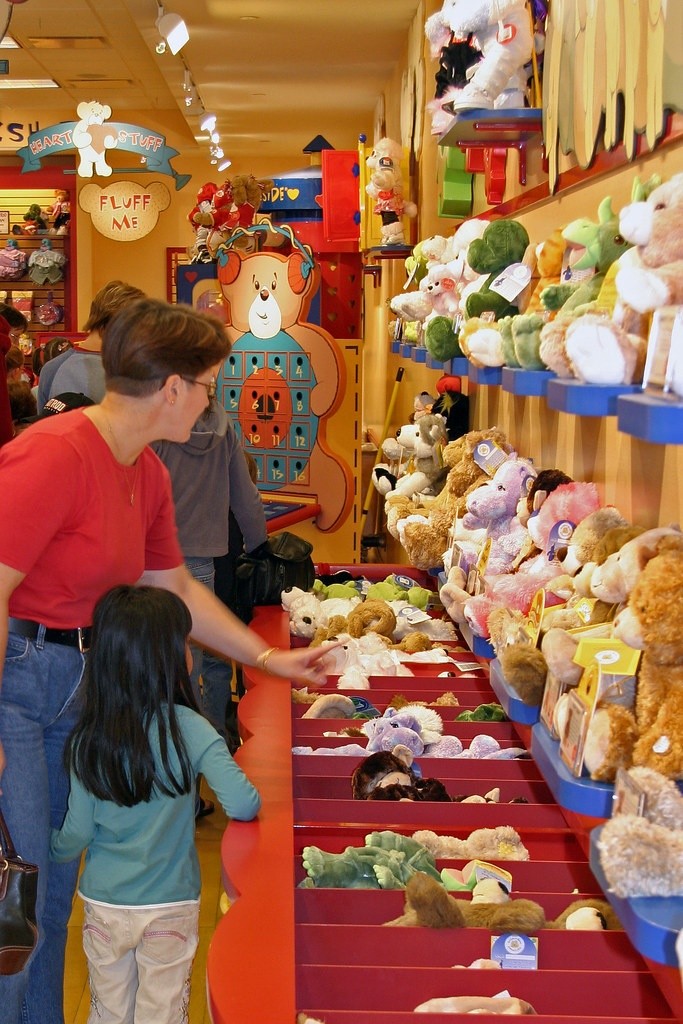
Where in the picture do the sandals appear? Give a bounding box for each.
[196,799,214,817]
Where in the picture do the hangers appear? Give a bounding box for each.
[40,238,51,250]
[7,237,17,252]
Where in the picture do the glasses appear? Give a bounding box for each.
[9,333,23,342]
[156,371,217,397]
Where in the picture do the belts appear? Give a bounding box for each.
[7,616,92,653]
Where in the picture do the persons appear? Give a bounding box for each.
[0,294,350,1024]
[48,583,262,1024]
[0,280,267,823]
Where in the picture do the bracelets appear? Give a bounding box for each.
[257,647,279,677]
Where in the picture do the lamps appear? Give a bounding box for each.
[157,7,190,55]
[217,159,231,171]
[195,108,216,130]
[214,144,224,158]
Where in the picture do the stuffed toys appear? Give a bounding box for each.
[424,0,549,136]
[12,189,70,236]
[281,392,683,1017]
[187,175,274,265]
[366,181,417,247]
[388,172,683,396]
[366,137,418,192]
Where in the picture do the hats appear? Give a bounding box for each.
[20,392,96,425]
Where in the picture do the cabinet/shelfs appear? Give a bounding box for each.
[0,154,91,372]
[289,340,683,1024]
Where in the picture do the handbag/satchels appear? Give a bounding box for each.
[236,531,315,604]
[0,808,40,975]
